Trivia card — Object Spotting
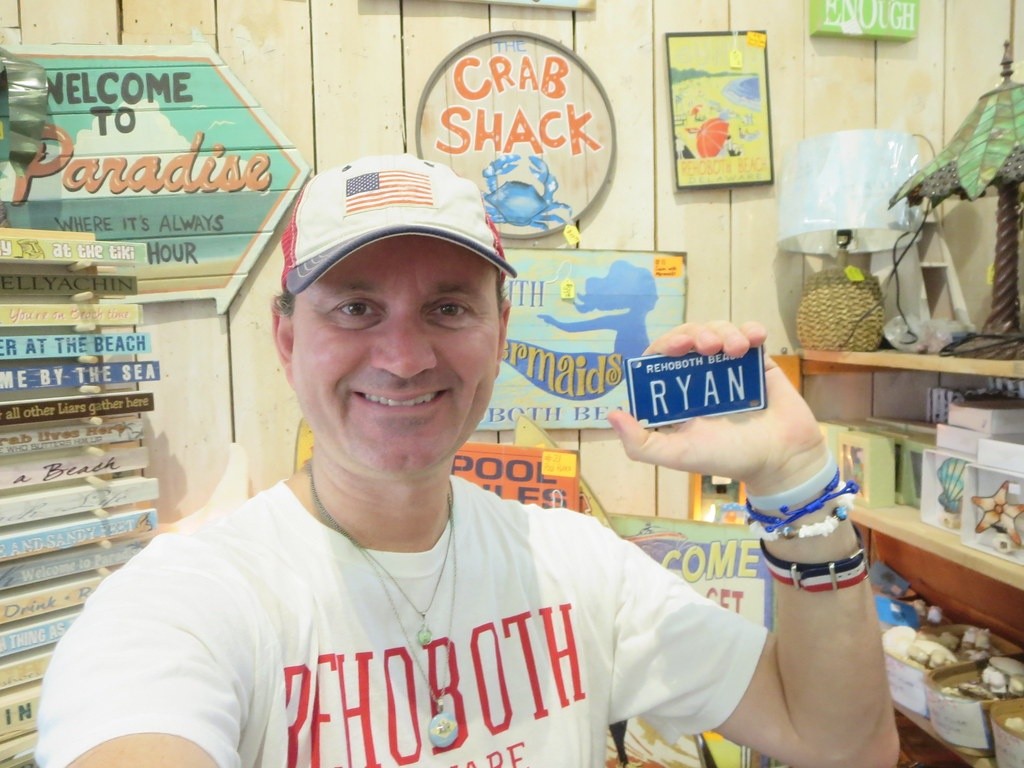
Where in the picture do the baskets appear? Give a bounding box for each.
[797,269,884,351]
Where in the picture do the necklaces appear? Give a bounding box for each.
[308,470,460,746]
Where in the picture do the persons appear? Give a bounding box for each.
[35,154,902,768]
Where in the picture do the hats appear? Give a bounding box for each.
[281,157,518,296]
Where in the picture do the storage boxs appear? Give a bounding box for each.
[870,584,916,600]
[987,699,1023,768]
[877,621,1023,716]
[929,652,1024,749]
[816,387,1023,566]
[874,600,952,638]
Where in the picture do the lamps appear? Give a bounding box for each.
[886,39,1024,362]
[773,131,925,352]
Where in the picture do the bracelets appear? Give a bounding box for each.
[742,453,856,540]
[758,529,871,592]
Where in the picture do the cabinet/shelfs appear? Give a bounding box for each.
[749,351,1023,768]
